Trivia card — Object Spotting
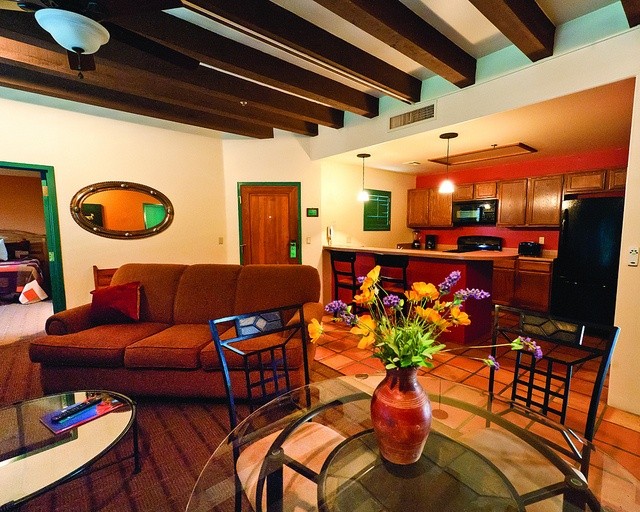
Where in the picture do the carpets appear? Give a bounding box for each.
[1,326,375,512]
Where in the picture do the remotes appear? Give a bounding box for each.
[50,395,101,422]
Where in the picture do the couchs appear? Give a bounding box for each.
[29,263,324,398]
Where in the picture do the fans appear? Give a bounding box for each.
[0,0,201,78]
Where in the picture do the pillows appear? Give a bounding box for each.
[90,280,141,321]
[0,239,8,262]
[15,250,29,260]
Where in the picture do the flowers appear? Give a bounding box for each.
[306,266,543,372]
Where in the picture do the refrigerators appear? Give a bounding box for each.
[551,195,625,327]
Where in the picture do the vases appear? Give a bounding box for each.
[370,363,433,464]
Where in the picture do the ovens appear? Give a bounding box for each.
[518,241,541,257]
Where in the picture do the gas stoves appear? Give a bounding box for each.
[441,235,503,253]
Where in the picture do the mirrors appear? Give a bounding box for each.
[69,181,175,240]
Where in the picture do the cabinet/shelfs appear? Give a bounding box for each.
[517,258,552,313]
[606,167,625,192]
[491,256,517,306]
[563,169,606,194]
[407,185,453,230]
[474,180,500,200]
[452,183,475,201]
[496,172,563,228]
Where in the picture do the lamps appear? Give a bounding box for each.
[31,1,112,79]
[436,132,458,196]
[355,153,371,202]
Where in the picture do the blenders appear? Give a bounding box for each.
[411,230,423,249]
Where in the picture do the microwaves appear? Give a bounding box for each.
[452,199,499,229]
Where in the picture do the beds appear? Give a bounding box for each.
[0,238,49,305]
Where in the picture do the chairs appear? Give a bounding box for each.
[486,302,621,480]
[207,303,312,512]
[329,251,366,327]
[373,252,413,324]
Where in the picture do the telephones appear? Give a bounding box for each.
[327,225,333,247]
